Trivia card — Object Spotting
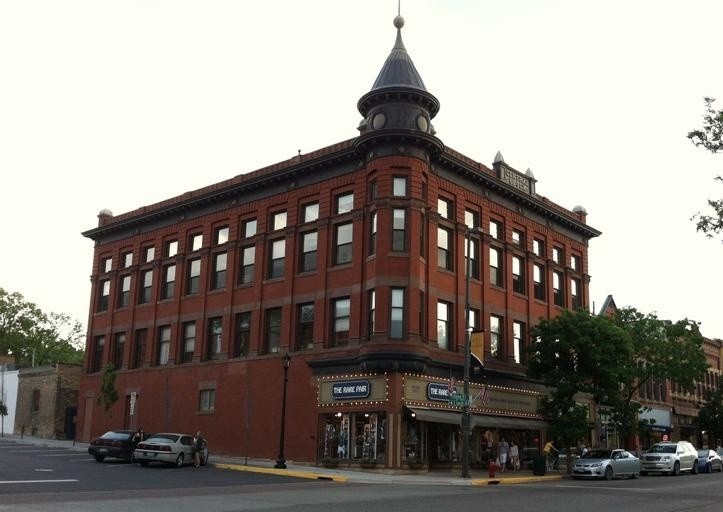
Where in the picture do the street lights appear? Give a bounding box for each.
[272,352,292,470]
[459,226,486,478]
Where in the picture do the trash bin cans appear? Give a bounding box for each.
[533,457,546,476]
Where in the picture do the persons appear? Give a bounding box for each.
[497,437,509,473]
[543,440,561,472]
[192,430,209,469]
[337,426,348,460]
[576,441,592,459]
[131,428,147,467]
[509,441,519,473]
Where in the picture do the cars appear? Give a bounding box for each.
[521,447,540,468]
[569,448,642,480]
[88,429,149,464]
[134,432,208,468]
[559,447,579,465]
[696,449,723,472]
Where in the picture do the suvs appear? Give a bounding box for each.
[639,440,699,476]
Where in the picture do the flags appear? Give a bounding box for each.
[448,369,454,394]
[481,387,489,406]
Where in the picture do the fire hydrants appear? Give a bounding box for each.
[487,458,497,478]
